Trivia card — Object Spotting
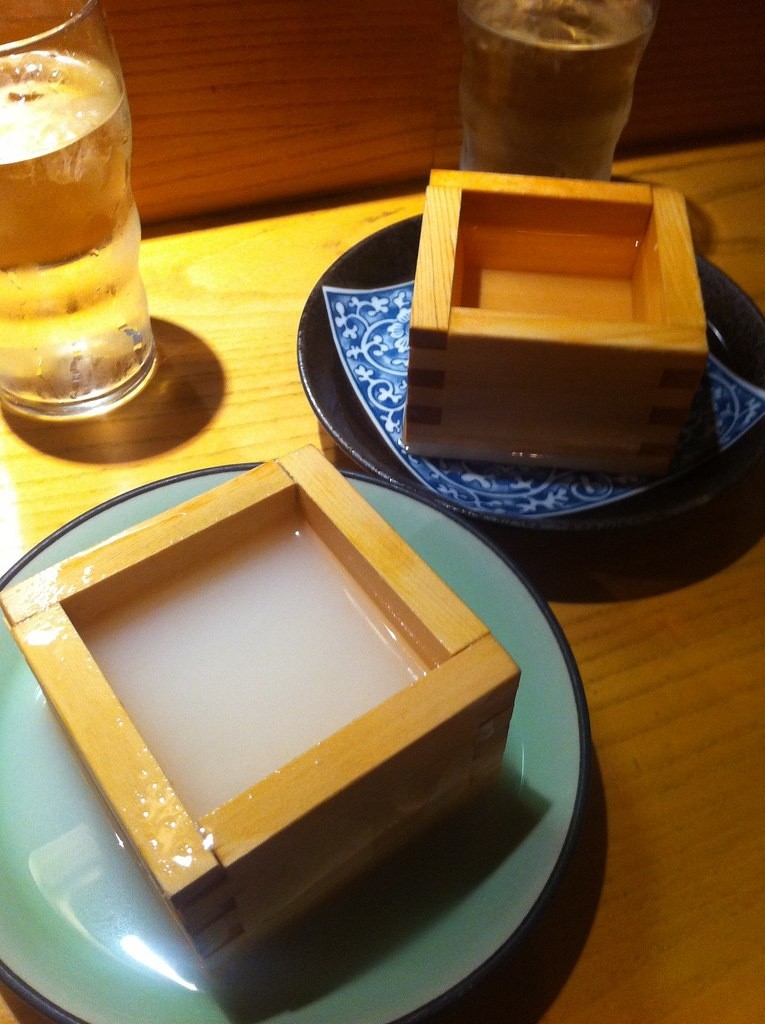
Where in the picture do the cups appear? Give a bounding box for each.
[0,0,159,424]
[453,1,657,181]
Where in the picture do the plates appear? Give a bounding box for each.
[0,463,589,1023]
[297,212,763,521]
